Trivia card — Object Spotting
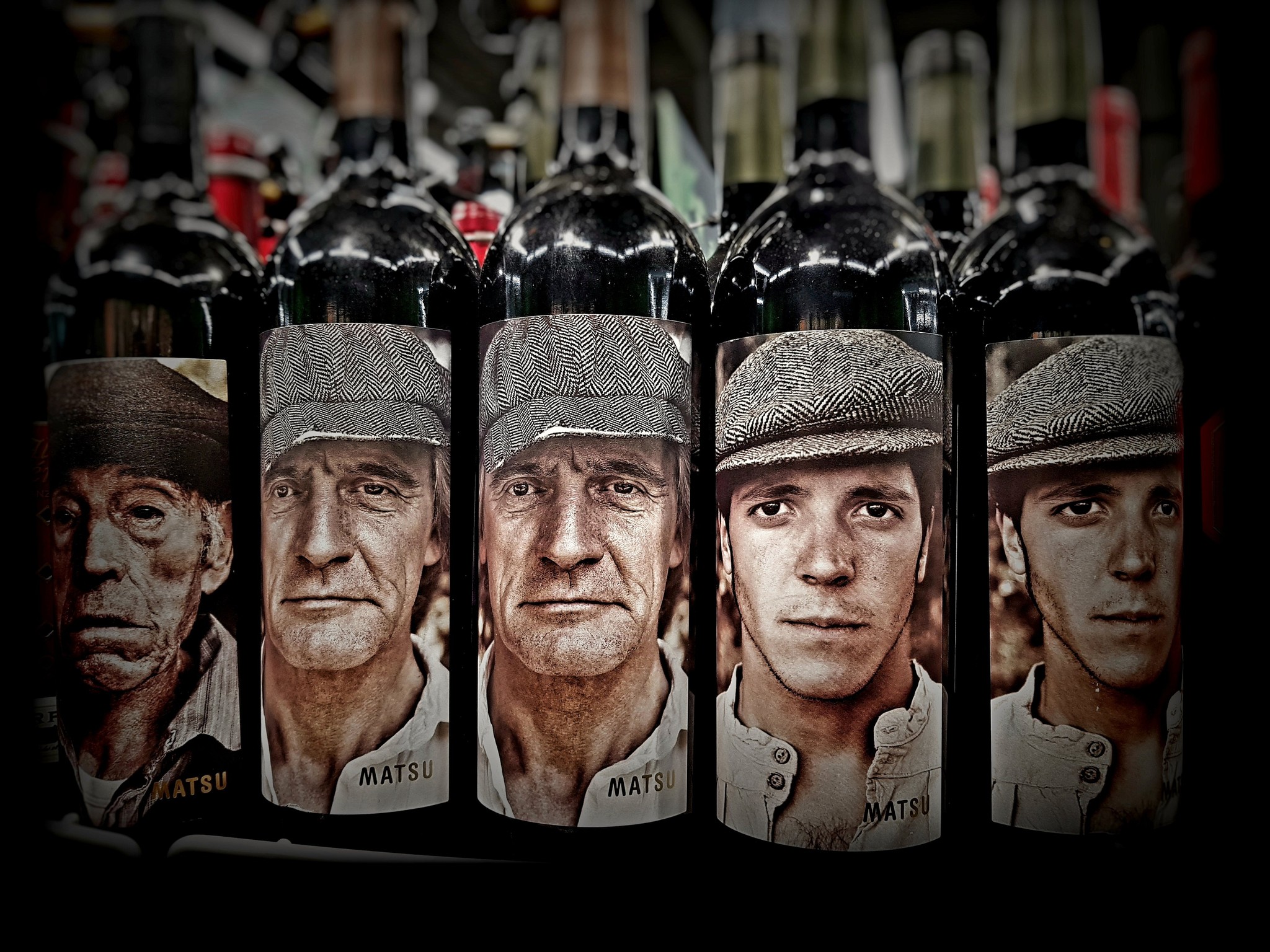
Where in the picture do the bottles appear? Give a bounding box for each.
[25,1,1221,879]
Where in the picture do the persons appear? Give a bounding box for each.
[259,323,452,813]
[478,315,692,833]
[987,335,1188,836]
[716,329,944,850]
[44,359,242,833]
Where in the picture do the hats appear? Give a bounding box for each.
[479,314,697,480]
[986,337,1183,480]
[717,328,947,471]
[254,321,448,474]
[43,356,231,482]
